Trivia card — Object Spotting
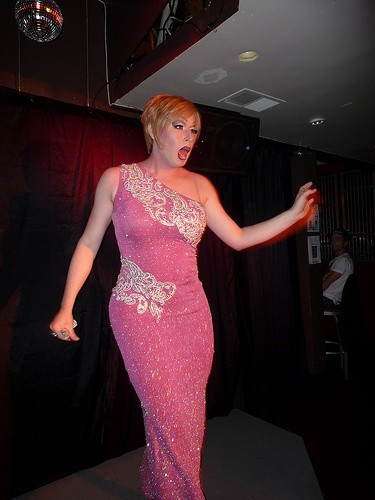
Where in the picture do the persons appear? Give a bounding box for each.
[318,228,355,308]
[48,93,318,500]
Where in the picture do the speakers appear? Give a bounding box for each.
[185,107,260,176]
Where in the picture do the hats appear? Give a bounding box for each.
[331,229,348,238]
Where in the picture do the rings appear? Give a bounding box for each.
[50,331,58,337]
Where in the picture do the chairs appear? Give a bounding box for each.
[320,271,366,371]
